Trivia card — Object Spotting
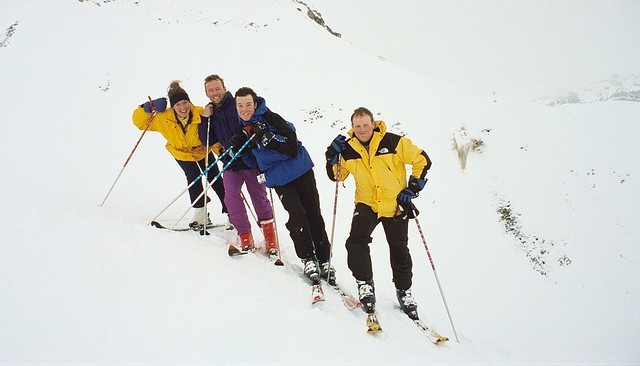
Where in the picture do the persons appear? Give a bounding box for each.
[229,86,337,285]
[325,107,432,321]
[197,74,280,257]
[132,80,235,230]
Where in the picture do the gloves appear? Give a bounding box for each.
[251,121,276,147]
[325,134,348,164]
[397,174,428,207]
[230,132,247,157]
[219,153,235,169]
[143,97,167,114]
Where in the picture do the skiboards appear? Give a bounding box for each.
[367,312,449,344]
[310,282,362,310]
[151,221,225,231]
[228,245,284,266]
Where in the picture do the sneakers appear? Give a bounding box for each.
[303,257,319,278]
[317,259,336,278]
[356,279,376,305]
[395,284,417,314]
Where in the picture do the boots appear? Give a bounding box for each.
[240,232,255,252]
[225,217,235,230]
[259,219,278,254]
[190,203,207,228]
